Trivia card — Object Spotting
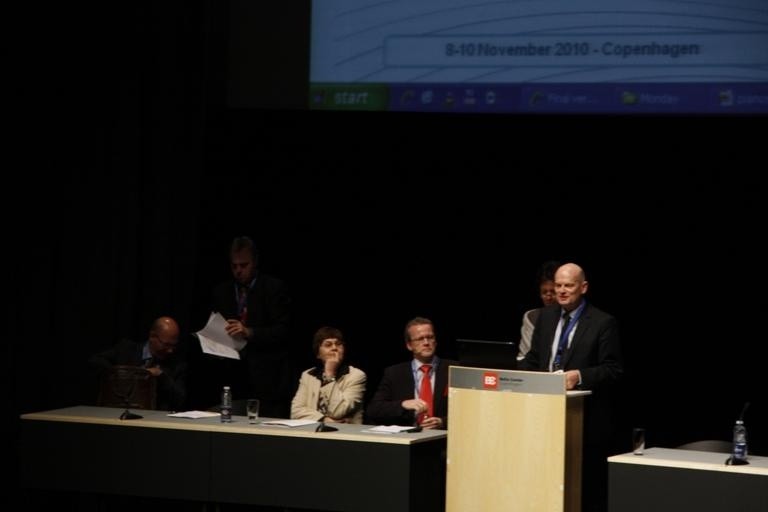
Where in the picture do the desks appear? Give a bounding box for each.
[607,446,768,512]
[21,405,447,510]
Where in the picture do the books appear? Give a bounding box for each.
[189,308,249,363]
[262,417,317,429]
[166,409,222,421]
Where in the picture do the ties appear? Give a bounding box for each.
[417,365,434,424]
[239,288,248,326]
[552,314,571,371]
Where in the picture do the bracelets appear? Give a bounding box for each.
[322,373,335,382]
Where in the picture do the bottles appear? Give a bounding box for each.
[220,384,232,422]
[732,418,749,460]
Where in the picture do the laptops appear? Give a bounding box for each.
[454,339,515,370]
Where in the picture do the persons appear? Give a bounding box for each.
[106,312,186,412]
[517,263,624,512]
[515,265,562,363]
[290,324,369,424]
[205,231,290,419]
[367,316,462,431]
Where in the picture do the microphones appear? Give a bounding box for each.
[315,363,354,433]
[723,399,761,465]
[118,356,160,420]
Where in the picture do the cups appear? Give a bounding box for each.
[246,398,260,423]
[632,426,644,456]
